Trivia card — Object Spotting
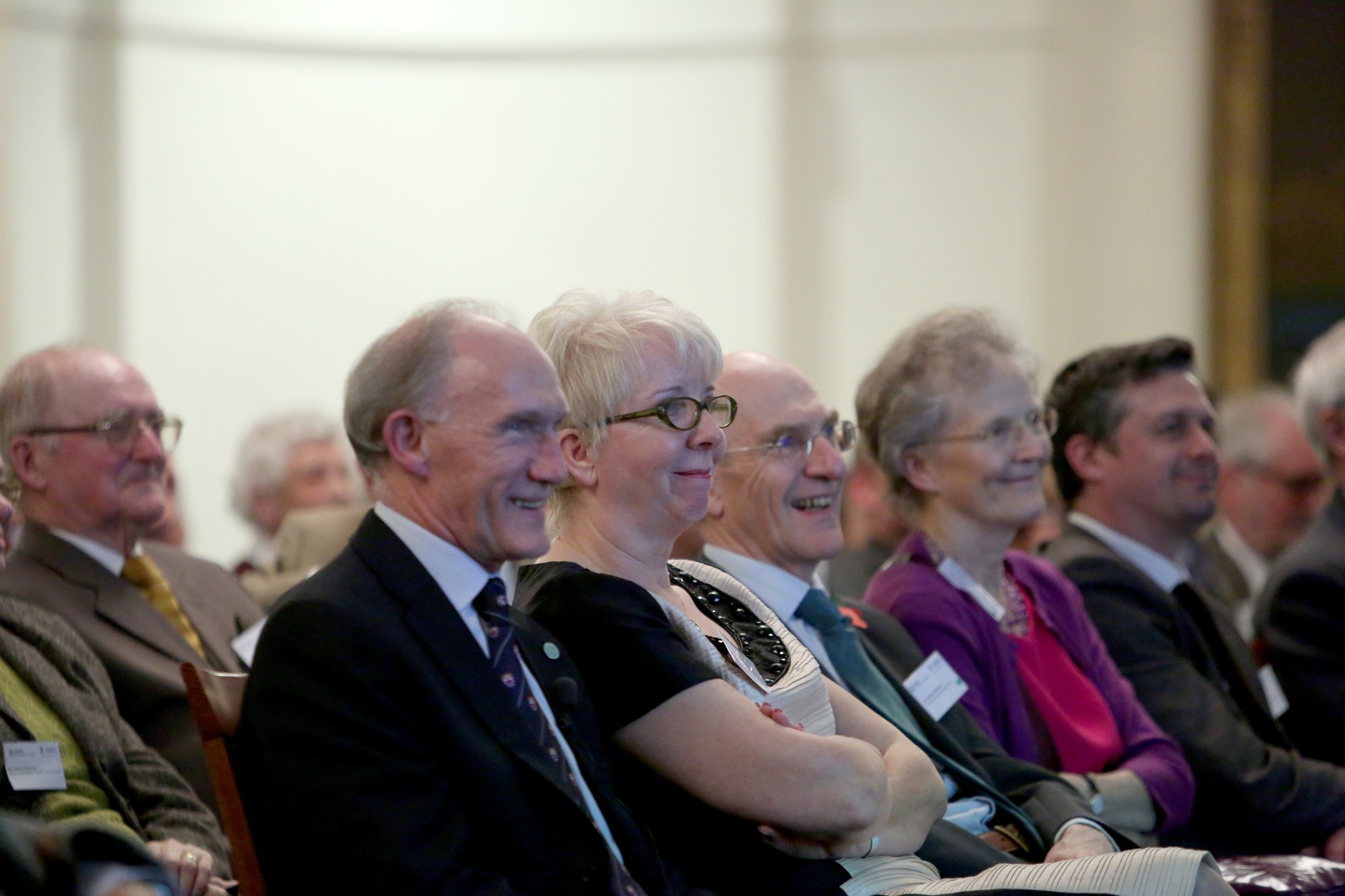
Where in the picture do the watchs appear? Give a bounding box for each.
[1081,773,1104,816]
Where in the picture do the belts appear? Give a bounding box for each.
[978,822,1028,853]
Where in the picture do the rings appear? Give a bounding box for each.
[180,853,198,862]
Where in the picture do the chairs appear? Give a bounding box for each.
[175,661,278,896]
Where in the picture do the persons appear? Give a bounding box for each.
[0,288,1344,896]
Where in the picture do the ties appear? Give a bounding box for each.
[471,577,642,896]
[795,588,1047,855]
[123,555,207,662]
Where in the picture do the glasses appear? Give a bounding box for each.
[571,394,739,430]
[903,406,1060,453]
[1235,460,1327,497]
[727,418,859,467]
[23,412,182,452]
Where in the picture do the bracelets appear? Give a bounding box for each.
[862,833,879,859]
